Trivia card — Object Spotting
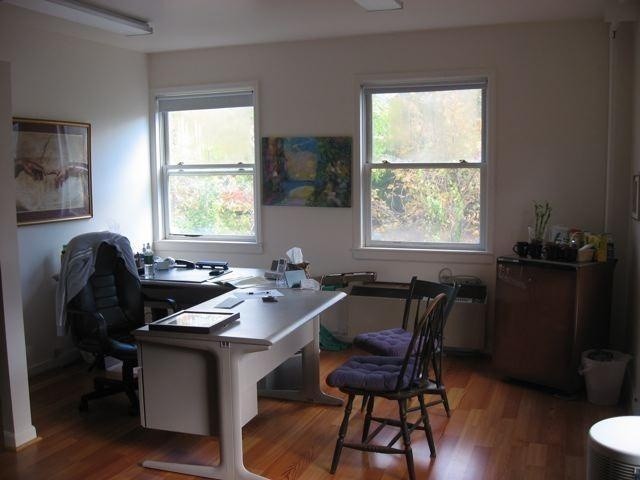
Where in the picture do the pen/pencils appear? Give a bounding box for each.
[249,291,269,294]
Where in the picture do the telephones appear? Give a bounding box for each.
[264,258,286,280]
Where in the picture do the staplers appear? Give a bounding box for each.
[174,259,195,268]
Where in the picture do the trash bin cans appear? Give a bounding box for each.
[581,348,633,406]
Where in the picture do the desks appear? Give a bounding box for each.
[137,262,268,311]
[130,288,347,480]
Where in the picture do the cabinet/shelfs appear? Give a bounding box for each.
[490,255,618,397]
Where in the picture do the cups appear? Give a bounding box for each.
[512,241,577,264]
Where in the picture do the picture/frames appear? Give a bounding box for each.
[11,116,94,226]
[630,175,640,220]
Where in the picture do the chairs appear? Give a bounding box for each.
[327,292,447,480]
[352,276,462,418]
[63,231,176,415]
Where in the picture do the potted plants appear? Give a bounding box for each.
[529,201,552,257]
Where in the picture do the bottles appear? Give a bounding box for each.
[143,245,154,281]
